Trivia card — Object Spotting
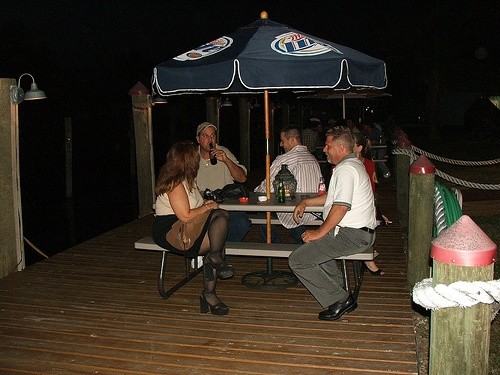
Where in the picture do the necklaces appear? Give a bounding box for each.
[202,155,212,167]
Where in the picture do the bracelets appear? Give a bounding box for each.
[205,203,209,210]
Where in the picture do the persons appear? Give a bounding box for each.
[157,115,393,319]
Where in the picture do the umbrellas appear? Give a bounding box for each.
[152,12,393,242]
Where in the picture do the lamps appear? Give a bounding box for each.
[147,94,168,106]
[273,98,281,109]
[10,74,47,104]
[218,96,233,108]
[249,97,261,111]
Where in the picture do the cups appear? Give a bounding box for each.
[239,196,248,205]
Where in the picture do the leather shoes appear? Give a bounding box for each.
[318,294,358,321]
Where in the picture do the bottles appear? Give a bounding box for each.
[273,165,297,198]
[285,185,291,200]
[277,178,285,203]
[318,176,326,196]
[209,143,217,165]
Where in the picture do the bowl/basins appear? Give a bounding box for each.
[258,196,267,202]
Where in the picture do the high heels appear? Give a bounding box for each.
[200,290,229,314]
[205,251,234,280]
[360,261,384,275]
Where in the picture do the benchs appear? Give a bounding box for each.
[135,216,375,300]
[319,159,386,164]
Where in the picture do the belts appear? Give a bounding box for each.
[360,227,375,234]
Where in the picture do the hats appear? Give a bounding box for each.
[197,122,217,135]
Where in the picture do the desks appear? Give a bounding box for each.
[317,144,386,156]
[186,193,365,286]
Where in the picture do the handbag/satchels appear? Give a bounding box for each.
[166,199,213,251]
